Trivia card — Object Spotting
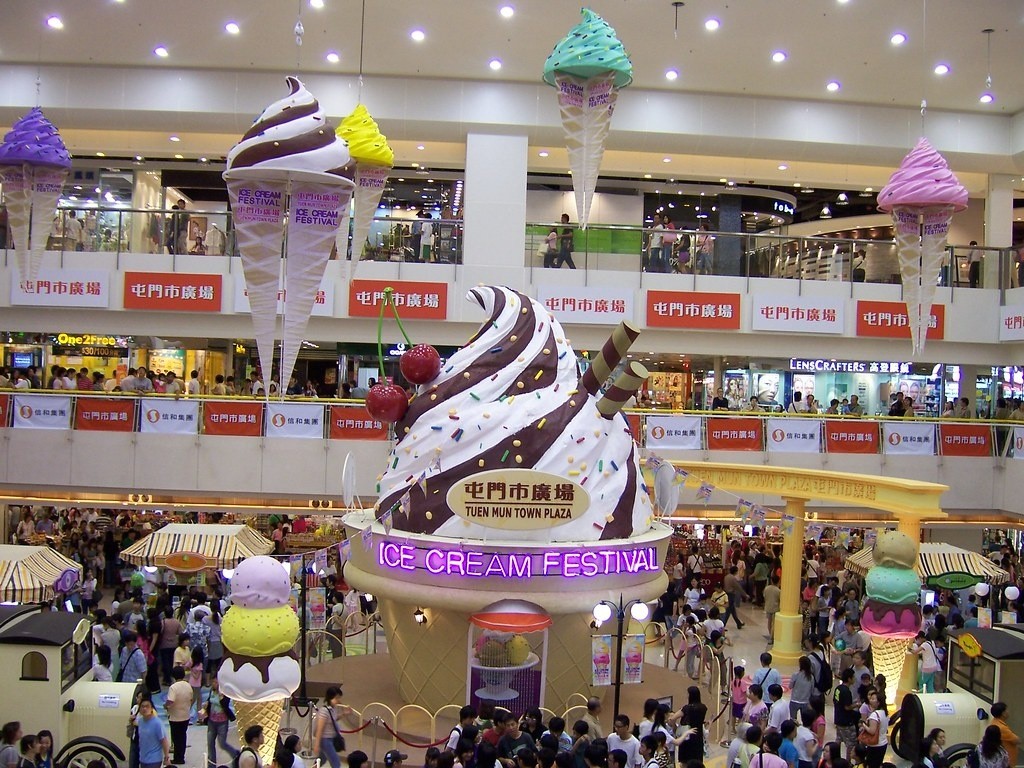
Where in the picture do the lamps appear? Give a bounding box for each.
[414,608,427,626]
[589,620,603,631]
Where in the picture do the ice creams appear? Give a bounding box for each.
[217,557,301,768]
[345,284,674,724]
[861,531,923,705]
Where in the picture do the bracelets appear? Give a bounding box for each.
[164,757,170,761]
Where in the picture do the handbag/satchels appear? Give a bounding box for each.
[310,637,318,656]
[858,711,880,745]
[935,655,942,671]
[333,734,345,752]
[664,223,677,242]
[696,249,701,260]
[537,240,549,256]
[93,589,103,602]
[127,724,134,737]
[730,758,741,768]
[116,668,123,681]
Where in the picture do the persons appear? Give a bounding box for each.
[543,226,558,268]
[641,213,715,275]
[49,197,209,255]
[853,248,866,282]
[420,524,1024,768]
[624,386,1023,420]
[0,363,381,399]
[1,504,410,768]
[555,214,577,269]
[1011,242,1024,287]
[411,210,433,263]
[704,373,926,412]
[967,241,983,288]
[940,248,951,286]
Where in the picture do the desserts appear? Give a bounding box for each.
[877,137,968,212]
[335,105,394,165]
[544,9,632,85]
[1,107,71,171]
[226,77,357,184]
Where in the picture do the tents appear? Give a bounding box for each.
[1,524,278,604]
[843,541,1010,616]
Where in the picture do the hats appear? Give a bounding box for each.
[416,209,422,216]
[748,541,755,547]
[384,750,408,762]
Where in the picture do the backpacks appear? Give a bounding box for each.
[232,747,258,768]
[810,645,832,695]
